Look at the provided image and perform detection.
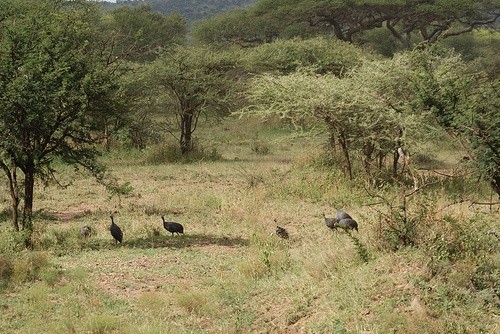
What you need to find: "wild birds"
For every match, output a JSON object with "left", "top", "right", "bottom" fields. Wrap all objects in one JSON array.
[
  {"left": 322, "top": 211, "right": 340, "bottom": 238},
  {"left": 109, "top": 215, "right": 123, "bottom": 245},
  {"left": 334, "top": 207, "right": 352, "bottom": 223},
  {"left": 274, "top": 218, "right": 289, "bottom": 241},
  {"left": 161, "top": 215, "right": 184, "bottom": 237},
  {"left": 334, "top": 218, "right": 358, "bottom": 236},
  {"left": 81, "top": 225, "right": 91, "bottom": 239}
]
